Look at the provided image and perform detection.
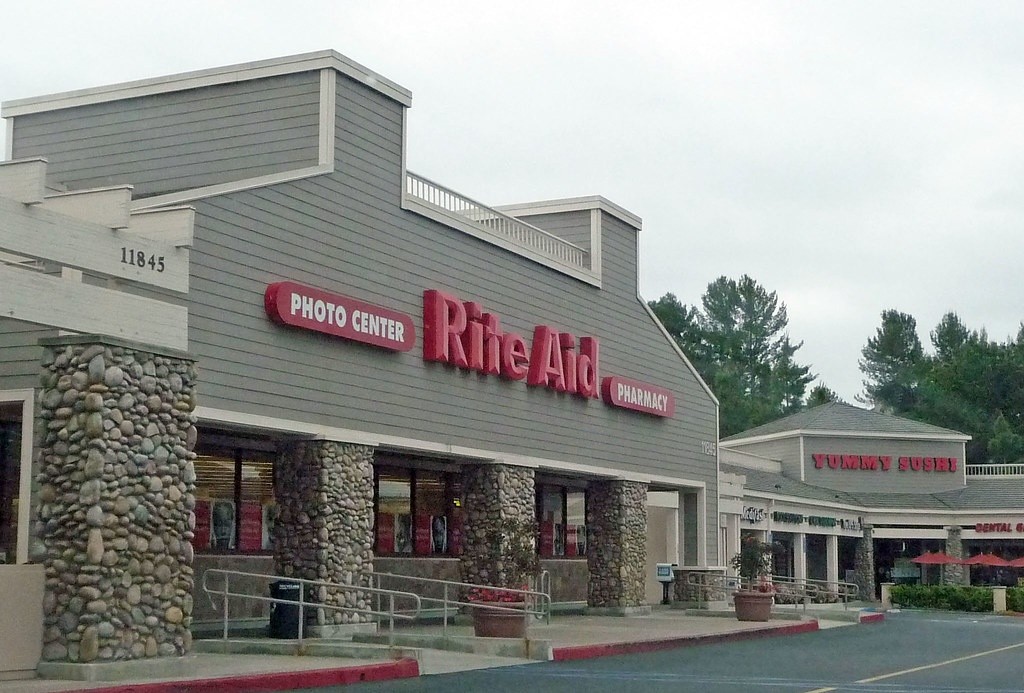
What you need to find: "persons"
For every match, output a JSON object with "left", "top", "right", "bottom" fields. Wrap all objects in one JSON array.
[
  {"left": 577, "top": 527, "right": 585, "bottom": 556},
  {"left": 212, "top": 502, "right": 234, "bottom": 549},
  {"left": 264, "top": 502, "right": 280, "bottom": 549},
  {"left": 554, "top": 524, "right": 564, "bottom": 554},
  {"left": 432, "top": 516, "right": 446, "bottom": 554},
  {"left": 396, "top": 514, "right": 411, "bottom": 551}
]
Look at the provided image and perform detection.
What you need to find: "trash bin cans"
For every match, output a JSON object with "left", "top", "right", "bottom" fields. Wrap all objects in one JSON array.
[{"left": 268, "top": 580, "right": 313, "bottom": 640}]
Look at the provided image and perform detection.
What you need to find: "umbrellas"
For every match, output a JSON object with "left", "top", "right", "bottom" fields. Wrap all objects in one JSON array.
[
  {"left": 961, "top": 554, "right": 1024, "bottom": 586},
  {"left": 910, "top": 551, "right": 962, "bottom": 584}
]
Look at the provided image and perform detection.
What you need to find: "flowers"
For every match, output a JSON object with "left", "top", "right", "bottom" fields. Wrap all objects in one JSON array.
[{"left": 470, "top": 582, "right": 530, "bottom": 602}]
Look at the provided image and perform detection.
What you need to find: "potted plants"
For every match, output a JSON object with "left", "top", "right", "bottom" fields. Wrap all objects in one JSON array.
[
  {"left": 473, "top": 517, "right": 542, "bottom": 638},
  {"left": 728, "top": 532, "right": 785, "bottom": 621}
]
